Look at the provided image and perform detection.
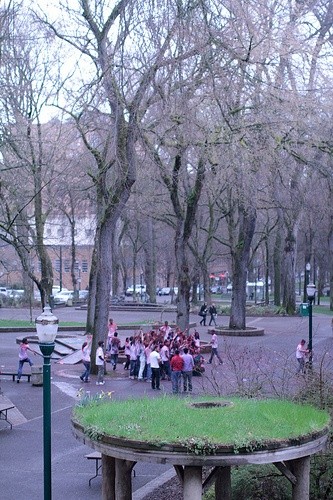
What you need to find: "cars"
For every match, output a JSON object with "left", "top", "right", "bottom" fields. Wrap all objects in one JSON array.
[
  {"left": 0, "top": 286, "right": 24, "bottom": 300},
  {"left": 158, "top": 287, "right": 179, "bottom": 296},
  {"left": 34, "top": 285, "right": 89, "bottom": 307}
]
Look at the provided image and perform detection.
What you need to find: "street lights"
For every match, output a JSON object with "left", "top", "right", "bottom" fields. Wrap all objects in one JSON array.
[
  {"left": 305, "top": 262, "right": 311, "bottom": 303},
  {"left": 306, "top": 280, "right": 316, "bottom": 377},
  {"left": 34, "top": 300, "right": 59, "bottom": 500}
]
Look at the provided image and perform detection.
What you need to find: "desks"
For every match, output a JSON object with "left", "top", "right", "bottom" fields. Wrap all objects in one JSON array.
[
  {"left": 85, "top": 450, "right": 135, "bottom": 486},
  {"left": 0, "top": 404, "right": 15, "bottom": 430}
]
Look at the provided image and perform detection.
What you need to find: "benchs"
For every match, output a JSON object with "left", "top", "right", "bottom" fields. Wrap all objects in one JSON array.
[{"left": 0, "top": 372, "right": 33, "bottom": 382}]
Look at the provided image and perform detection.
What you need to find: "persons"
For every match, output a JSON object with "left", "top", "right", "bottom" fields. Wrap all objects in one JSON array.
[
  {"left": 124, "top": 321, "right": 205, "bottom": 382},
  {"left": 200, "top": 302, "right": 208, "bottom": 326},
  {"left": 96, "top": 340, "right": 105, "bottom": 385},
  {"left": 16, "top": 338, "right": 37, "bottom": 383},
  {"left": 80, "top": 337, "right": 92, "bottom": 383},
  {"left": 149, "top": 346, "right": 162, "bottom": 390},
  {"left": 181, "top": 348, "right": 194, "bottom": 391},
  {"left": 209, "top": 303, "right": 217, "bottom": 326},
  {"left": 107, "top": 319, "right": 118, "bottom": 351},
  {"left": 170, "top": 351, "right": 185, "bottom": 395},
  {"left": 110, "top": 331, "right": 121, "bottom": 371},
  {"left": 295, "top": 339, "right": 309, "bottom": 375},
  {"left": 207, "top": 329, "right": 223, "bottom": 365}
]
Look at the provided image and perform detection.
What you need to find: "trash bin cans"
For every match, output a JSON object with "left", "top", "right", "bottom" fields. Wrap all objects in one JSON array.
[
  {"left": 299, "top": 303, "right": 309, "bottom": 316},
  {"left": 30, "top": 365, "right": 43, "bottom": 386}
]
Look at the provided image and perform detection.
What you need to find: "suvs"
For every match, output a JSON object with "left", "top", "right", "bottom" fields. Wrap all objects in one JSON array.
[{"left": 126, "top": 285, "right": 146, "bottom": 296}]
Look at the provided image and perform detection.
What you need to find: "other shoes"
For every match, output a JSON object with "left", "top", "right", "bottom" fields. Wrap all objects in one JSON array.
[
  {"left": 80, "top": 376, "right": 84, "bottom": 381},
  {"left": 17, "top": 379, "right": 20, "bottom": 383}
]
